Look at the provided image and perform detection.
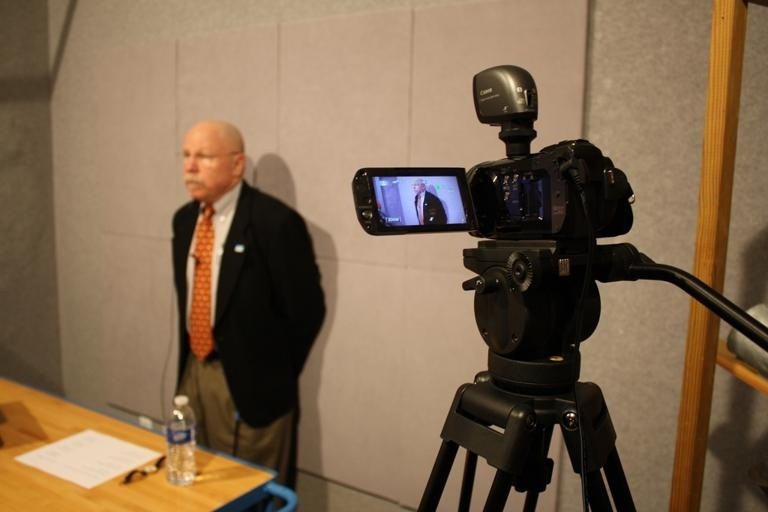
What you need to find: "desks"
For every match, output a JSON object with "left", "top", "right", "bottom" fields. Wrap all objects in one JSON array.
[{"left": 0, "top": 377, "right": 300, "bottom": 512}]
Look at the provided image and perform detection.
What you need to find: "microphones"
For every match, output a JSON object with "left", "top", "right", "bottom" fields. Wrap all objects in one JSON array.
[{"left": 193, "top": 255, "right": 200, "bottom": 267}]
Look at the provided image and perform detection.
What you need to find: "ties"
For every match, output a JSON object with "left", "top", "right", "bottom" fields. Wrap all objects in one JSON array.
[
  {"left": 417, "top": 195, "right": 423, "bottom": 225},
  {"left": 189, "top": 204, "right": 215, "bottom": 365}
]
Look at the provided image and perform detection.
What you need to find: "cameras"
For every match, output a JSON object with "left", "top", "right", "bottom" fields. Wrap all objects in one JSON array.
[{"left": 352, "top": 64, "right": 636, "bottom": 238}]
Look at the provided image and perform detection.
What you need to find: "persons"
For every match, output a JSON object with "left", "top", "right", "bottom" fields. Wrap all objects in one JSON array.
[
  {"left": 412, "top": 180, "right": 448, "bottom": 226},
  {"left": 162, "top": 118, "right": 330, "bottom": 496}
]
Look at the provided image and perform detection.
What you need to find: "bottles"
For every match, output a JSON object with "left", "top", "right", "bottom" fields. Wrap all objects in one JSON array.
[{"left": 166, "top": 394, "right": 196, "bottom": 487}]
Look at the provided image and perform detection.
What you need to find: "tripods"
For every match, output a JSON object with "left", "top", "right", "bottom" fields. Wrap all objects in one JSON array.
[{"left": 416, "top": 238, "right": 768, "bottom": 512}]
